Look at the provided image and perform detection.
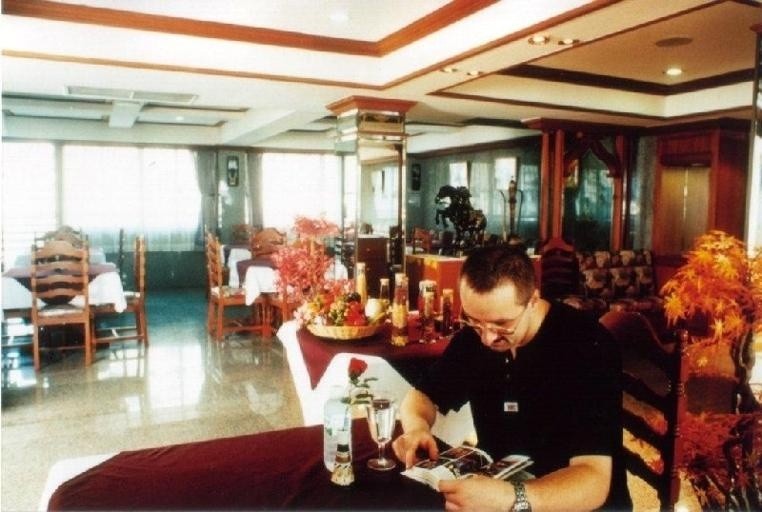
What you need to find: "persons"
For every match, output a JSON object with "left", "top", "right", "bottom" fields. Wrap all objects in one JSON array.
[{"left": 391, "top": 243, "right": 634, "bottom": 512}]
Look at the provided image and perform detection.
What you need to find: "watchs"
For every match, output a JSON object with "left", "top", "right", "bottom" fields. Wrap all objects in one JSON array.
[{"left": 507, "top": 480, "right": 533, "bottom": 511}]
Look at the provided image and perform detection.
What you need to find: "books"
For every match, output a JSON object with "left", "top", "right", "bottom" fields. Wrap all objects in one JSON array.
[{"left": 400, "top": 443, "right": 535, "bottom": 494}]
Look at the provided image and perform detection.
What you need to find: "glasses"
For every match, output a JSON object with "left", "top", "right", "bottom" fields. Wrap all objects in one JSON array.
[{"left": 457, "top": 297, "right": 524, "bottom": 334}]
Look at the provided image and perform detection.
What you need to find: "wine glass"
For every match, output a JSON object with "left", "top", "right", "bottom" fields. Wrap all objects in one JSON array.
[{"left": 362, "top": 393, "right": 398, "bottom": 472}]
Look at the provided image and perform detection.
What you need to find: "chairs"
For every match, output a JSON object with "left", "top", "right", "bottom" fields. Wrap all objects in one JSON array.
[
  {"left": 2, "top": 277, "right": 33, "bottom": 356},
  {"left": 34, "top": 228, "right": 90, "bottom": 260},
  {"left": 31, "top": 245, "right": 96, "bottom": 369},
  {"left": 605, "top": 310, "right": 680, "bottom": 511},
  {"left": 200, "top": 223, "right": 761, "bottom": 413},
  {"left": 92, "top": 238, "right": 147, "bottom": 353}
]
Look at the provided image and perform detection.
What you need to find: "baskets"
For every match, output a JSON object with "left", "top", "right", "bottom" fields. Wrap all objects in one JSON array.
[{"left": 307, "top": 324, "right": 377, "bottom": 340}]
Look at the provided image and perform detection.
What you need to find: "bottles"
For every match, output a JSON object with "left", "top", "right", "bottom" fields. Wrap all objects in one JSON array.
[
  {"left": 350, "top": 260, "right": 454, "bottom": 347},
  {"left": 322, "top": 385, "right": 354, "bottom": 473}
]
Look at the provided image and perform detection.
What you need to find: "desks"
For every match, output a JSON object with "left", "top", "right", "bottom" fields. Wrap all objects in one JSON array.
[
  {"left": 6, "top": 264, "right": 121, "bottom": 353},
  {"left": 46, "top": 417, "right": 452, "bottom": 510}
]
